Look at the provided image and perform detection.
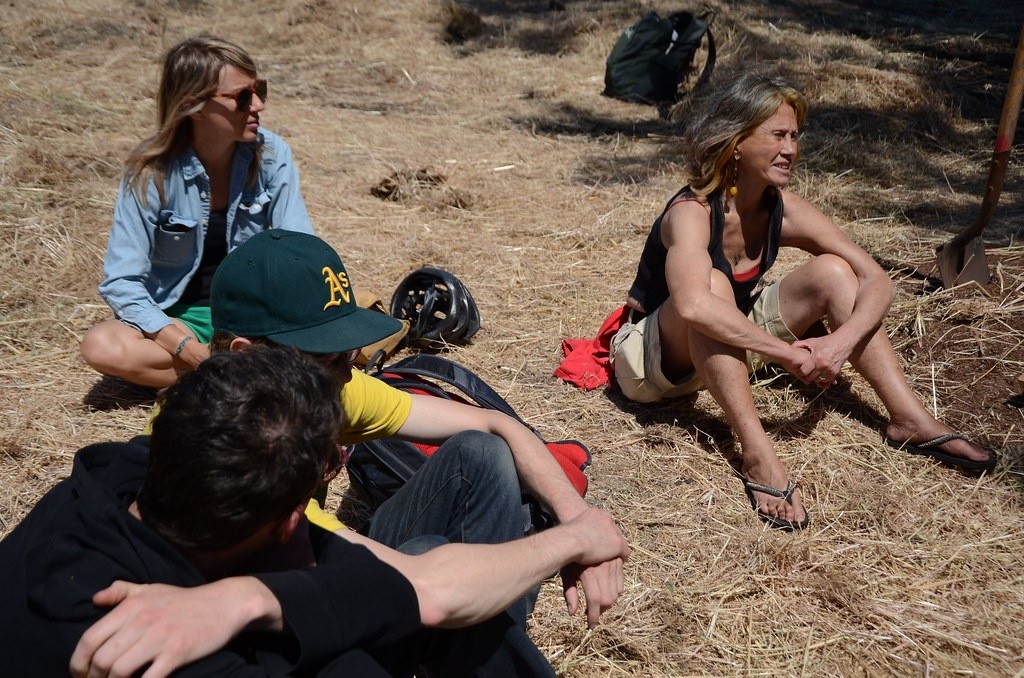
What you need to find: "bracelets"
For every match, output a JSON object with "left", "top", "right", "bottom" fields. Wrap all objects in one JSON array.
[{"left": 176, "top": 336, "right": 193, "bottom": 356}]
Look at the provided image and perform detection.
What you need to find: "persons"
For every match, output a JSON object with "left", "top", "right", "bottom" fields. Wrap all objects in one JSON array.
[
  {"left": 0, "top": 345, "right": 421, "bottom": 678},
  {"left": 134, "top": 228, "right": 632, "bottom": 678},
  {"left": 608, "top": 69, "right": 996, "bottom": 528},
  {"left": 80, "top": 32, "right": 318, "bottom": 395}
]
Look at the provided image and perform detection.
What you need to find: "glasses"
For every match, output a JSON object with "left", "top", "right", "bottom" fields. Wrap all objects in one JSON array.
[{"left": 206, "top": 79, "right": 268, "bottom": 111}]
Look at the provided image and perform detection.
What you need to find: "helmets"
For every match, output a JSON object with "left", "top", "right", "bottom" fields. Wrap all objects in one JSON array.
[{"left": 390, "top": 265, "right": 479, "bottom": 352}]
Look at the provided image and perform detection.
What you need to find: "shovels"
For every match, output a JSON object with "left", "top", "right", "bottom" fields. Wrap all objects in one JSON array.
[{"left": 935, "top": 23, "right": 1024, "bottom": 293}]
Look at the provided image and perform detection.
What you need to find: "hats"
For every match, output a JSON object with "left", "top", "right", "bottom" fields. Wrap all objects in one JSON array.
[{"left": 211, "top": 229, "right": 403, "bottom": 352}]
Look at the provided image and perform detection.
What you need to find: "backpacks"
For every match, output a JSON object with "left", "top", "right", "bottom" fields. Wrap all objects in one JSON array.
[
  {"left": 599, "top": 10, "right": 716, "bottom": 106},
  {"left": 346, "top": 355, "right": 592, "bottom": 518}
]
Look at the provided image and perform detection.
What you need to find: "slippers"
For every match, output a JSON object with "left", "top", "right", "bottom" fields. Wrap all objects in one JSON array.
[
  {"left": 741, "top": 474, "right": 809, "bottom": 532},
  {"left": 883, "top": 434, "right": 997, "bottom": 476}
]
